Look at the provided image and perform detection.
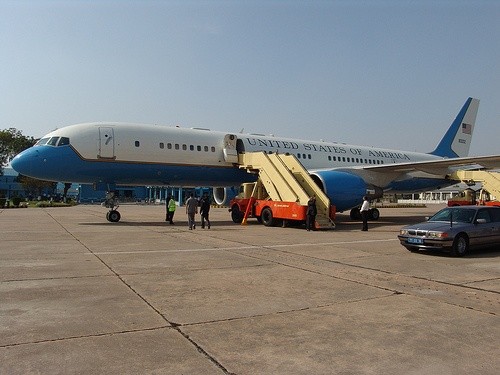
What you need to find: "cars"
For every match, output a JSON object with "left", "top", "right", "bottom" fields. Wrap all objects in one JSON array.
[{"left": 397, "top": 204, "right": 500, "bottom": 257}]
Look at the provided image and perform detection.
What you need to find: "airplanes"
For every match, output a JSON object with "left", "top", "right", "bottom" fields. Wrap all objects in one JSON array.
[{"left": 9, "top": 97, "right": 500, "bottom": 235}]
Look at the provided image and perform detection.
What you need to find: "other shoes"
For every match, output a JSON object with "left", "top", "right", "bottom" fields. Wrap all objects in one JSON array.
[
  {"left": 189, "top": 226, "right": 192, "bottom": 230},
  {"left": 201, "top": 226, "right": 205, "bottom": 229},
  {"left": 307, "top": 228, "right": 310, "bottom": 231},
  {"left": 208, "top": 226, "right": 210, "bottom": 229},
  {"left": 193, "top": 225, "right": 195, "bottom": 229},
  {"left": 361, "top": 229, "right": 368, "bottom": 231},
  {"left": 313, "top": 229, "right": 317, "bottom": 231}
]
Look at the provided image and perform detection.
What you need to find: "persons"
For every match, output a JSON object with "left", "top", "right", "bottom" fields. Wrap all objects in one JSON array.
[
  {"left": 168, "top": 196, "right": 175, "bottom": 224},
  {"left": 186, "top": 193, "right": 198, "bottom": 230},
  {"left": 185, "top": 193, "right": 208, "bottom": 206},
  {"left": 307, "top": 197, "right": 317, "bottom": 231},
  {"left": 359, "top": 196, "right": 369, "bottom": 232},
  {"left": 200, "top": 199, "right": 210, "bottom": 229},
  {"left": 165, "top": 195, "right": 171, "bottom": 221},
  {"left": 36, "top": 191, "right": 65, "bottom": 202}
]
